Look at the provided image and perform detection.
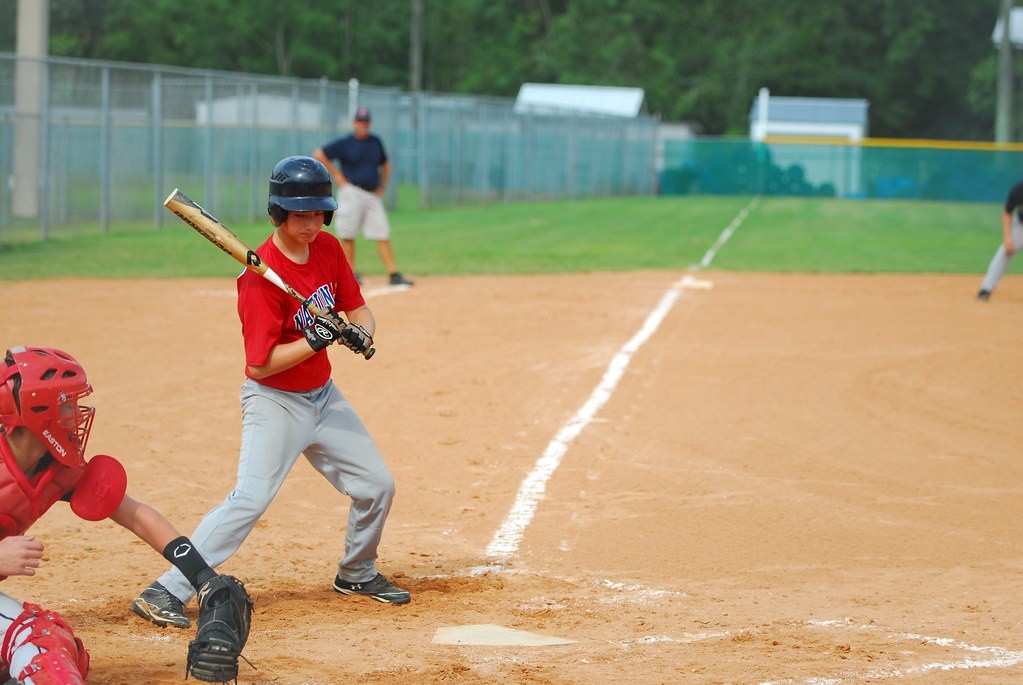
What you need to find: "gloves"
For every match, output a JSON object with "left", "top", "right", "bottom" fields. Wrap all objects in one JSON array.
[
  {"left": 337, "top": 322, "right": 374, "bottom": 355},
  {"left": 304, "top": 305, "right": 348, "bottom": 353}
]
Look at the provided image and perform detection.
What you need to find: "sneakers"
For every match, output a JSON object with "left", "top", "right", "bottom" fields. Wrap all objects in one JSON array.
[
  {"left": 131, "top": 583, "right": 190, "bottom": 628},
  {"left": 332, "top": 572, "right": 410, "bottom": 603}
]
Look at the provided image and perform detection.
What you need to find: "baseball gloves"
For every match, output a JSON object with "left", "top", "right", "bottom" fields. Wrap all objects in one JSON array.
[{"left": 183, "top": 566, "right": 259, "bottom": 685}]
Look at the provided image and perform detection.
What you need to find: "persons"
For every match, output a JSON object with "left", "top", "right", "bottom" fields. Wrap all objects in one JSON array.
[
  {"left": 132, "top": 154, "right": 411, "bottom": 629},
  {"left": 313, "top": 104, "right": 414, "bottom": 287},
  {"left": 0, "top": 341, "right": 253, "bottom": 685},
  {"left": 976, "top": 177, "right": 1023, "bottom": 302}
]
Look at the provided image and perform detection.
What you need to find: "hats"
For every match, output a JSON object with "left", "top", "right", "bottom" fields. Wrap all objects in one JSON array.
[{"left": 356, "top": 108, "right": 370, "bottom": 120}]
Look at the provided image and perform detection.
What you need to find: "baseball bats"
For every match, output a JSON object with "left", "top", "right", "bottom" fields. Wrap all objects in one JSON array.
[{"left": 163, "top": 186, "right": 377, "bottom": 361}]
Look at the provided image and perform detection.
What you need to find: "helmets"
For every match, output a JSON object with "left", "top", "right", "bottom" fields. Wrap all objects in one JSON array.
[
  {"left": 0, "top": 346, "right": 95, "bottom": 467},
  {"left": 268, "top": 156, "right": 339, "bottom": 227}
]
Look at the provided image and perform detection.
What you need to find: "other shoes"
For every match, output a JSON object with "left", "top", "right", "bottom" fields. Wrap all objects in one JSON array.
[
  {"left": 979, "top": 290, "right": 989, "bottom": 299},
  {"left": 389, "top": 275, "right": 412, "bottom": 285},
  {"left": 353, "top": 272, "right": 362, "bottom": 285}
]
